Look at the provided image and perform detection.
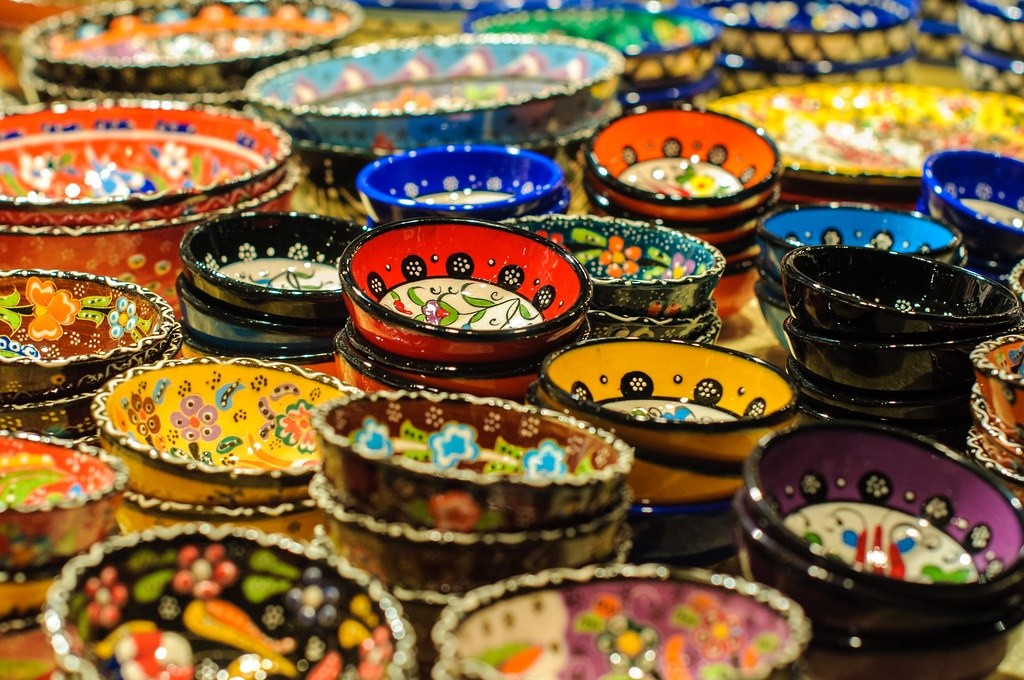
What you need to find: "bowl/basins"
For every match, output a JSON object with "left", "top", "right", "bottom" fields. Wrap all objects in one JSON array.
[{"left": 0, "top": 0, "right": 1024, "bottom": 680}]
